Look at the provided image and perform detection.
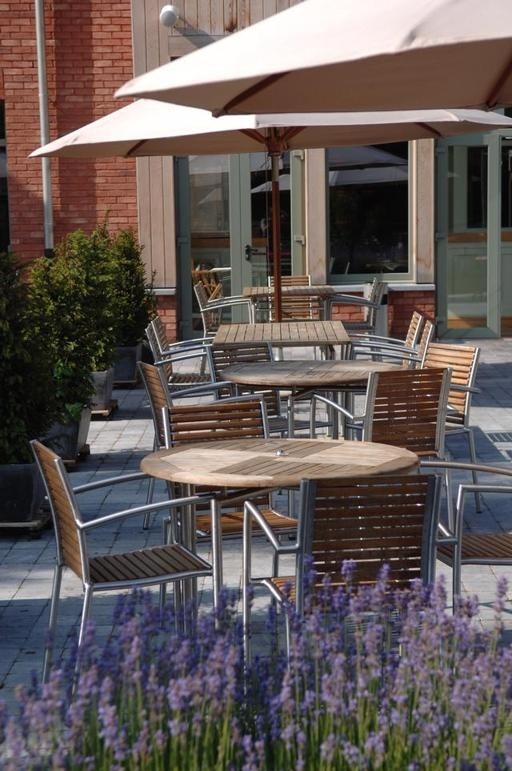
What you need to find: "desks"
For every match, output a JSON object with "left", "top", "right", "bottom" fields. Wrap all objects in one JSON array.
[{"left": 140, "top": 435, "right": 418, "bottom": 658}]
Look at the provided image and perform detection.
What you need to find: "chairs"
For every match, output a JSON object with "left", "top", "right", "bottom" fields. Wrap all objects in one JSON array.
[
  {"left": 161, "top": 400, "right": 298, "bottom": 639},
  {"left": 137, "top": 264, "right": 481, "bottom": 527},
  {"left": 29, "top": 440, "right": 216, "bottom": 706},
  {"left": 419, "top": 461, "right": 512, "bottom": 620},
  {"left": 238, "top": 473, "right": 444, "bottom": 702}
]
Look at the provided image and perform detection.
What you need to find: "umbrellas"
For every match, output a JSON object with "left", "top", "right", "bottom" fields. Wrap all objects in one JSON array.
[
  {"left": 189, "top": 144, "right": 457, "bottom": 209},
  {"left": 27, "top": 98, "right": 511, "bottom": 362},
  {"left": 115, "top": 0, "right": 512, "bottom": 113}
]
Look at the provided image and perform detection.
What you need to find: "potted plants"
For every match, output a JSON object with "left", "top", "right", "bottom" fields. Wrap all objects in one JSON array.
[{"left": 0, "top": 206, "right": 160, "bottom": 523}]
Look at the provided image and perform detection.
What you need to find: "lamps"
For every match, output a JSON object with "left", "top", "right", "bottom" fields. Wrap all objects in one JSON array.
[{"left": 160, "top": 5, "right": 178, "bottom": 28}]
